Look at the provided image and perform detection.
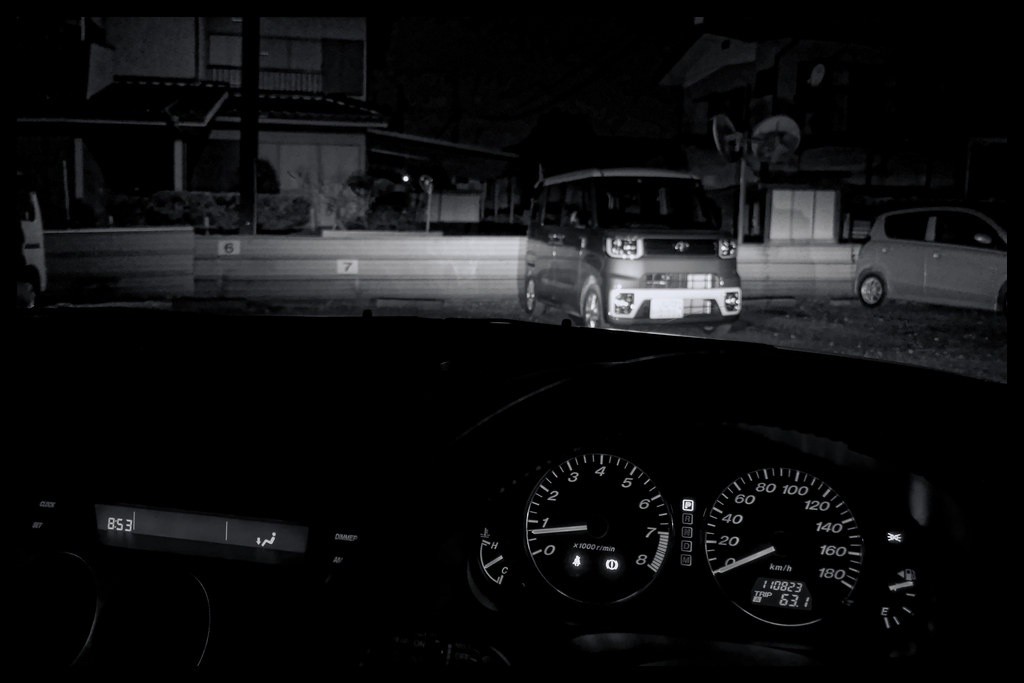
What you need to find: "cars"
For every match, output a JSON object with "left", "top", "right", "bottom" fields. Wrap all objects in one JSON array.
[{"left": 852, "top": 202, "right": 1008, "bottom": 319}]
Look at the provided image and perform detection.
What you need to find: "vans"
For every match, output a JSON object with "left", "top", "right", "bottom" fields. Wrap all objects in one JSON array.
[{"left": 521, "top": 167, "right": 748, "bottom": 333}]
